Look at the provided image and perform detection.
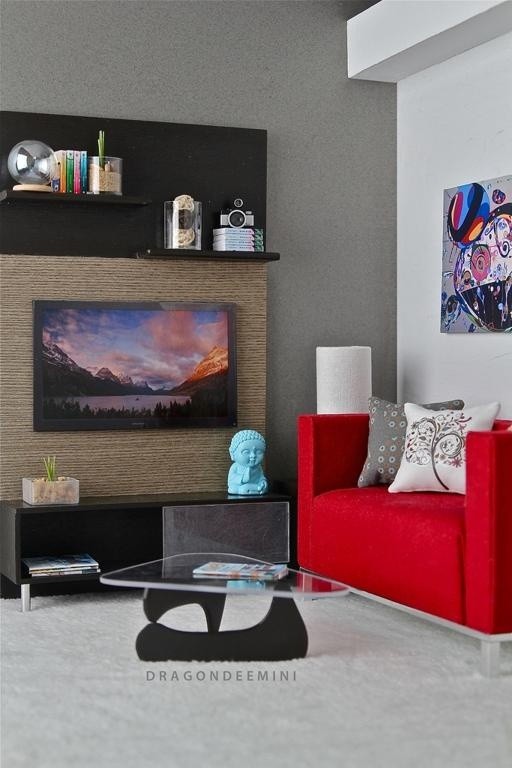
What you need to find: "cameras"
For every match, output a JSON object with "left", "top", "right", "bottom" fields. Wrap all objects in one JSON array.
[{"left": 219, "top": 199, "right": 255, "bottom": 228}]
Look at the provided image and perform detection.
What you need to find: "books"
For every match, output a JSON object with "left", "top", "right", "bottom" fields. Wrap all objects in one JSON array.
[
  {"left": 212, "top": 226, "right": 265, "bottom": 252},
  {"left": 49, "top": 149, "right": 89, "bottom": 194},
  {"left": 193, "top": 561, "right": 288, "bottom": 578},
  {"left": 22, "top": 552, "right": 103, "bottom": 578}
]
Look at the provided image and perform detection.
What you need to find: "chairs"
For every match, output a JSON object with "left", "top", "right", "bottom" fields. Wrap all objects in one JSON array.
[{"left": 298, "top": 413, "right": 512, "bottom": 634}]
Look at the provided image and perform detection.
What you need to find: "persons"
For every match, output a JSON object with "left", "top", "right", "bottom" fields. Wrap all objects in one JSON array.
[{"left": 227, "top": 429, "right": 268, "bottom": 495}]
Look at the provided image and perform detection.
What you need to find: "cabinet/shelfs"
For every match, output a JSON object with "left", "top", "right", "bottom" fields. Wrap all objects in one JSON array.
[{"left": 0, "top": 111, "right": 296, "bottom": 612}]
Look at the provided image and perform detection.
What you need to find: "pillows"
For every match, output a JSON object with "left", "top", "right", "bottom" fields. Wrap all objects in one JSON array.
[{"left": 356, "top": 397, "right": 501, "bottom": 496}]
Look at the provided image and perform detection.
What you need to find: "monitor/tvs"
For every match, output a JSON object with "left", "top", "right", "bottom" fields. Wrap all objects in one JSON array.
[{"left": 32, "top": 300, "right": 236, "bottom": 431}]
